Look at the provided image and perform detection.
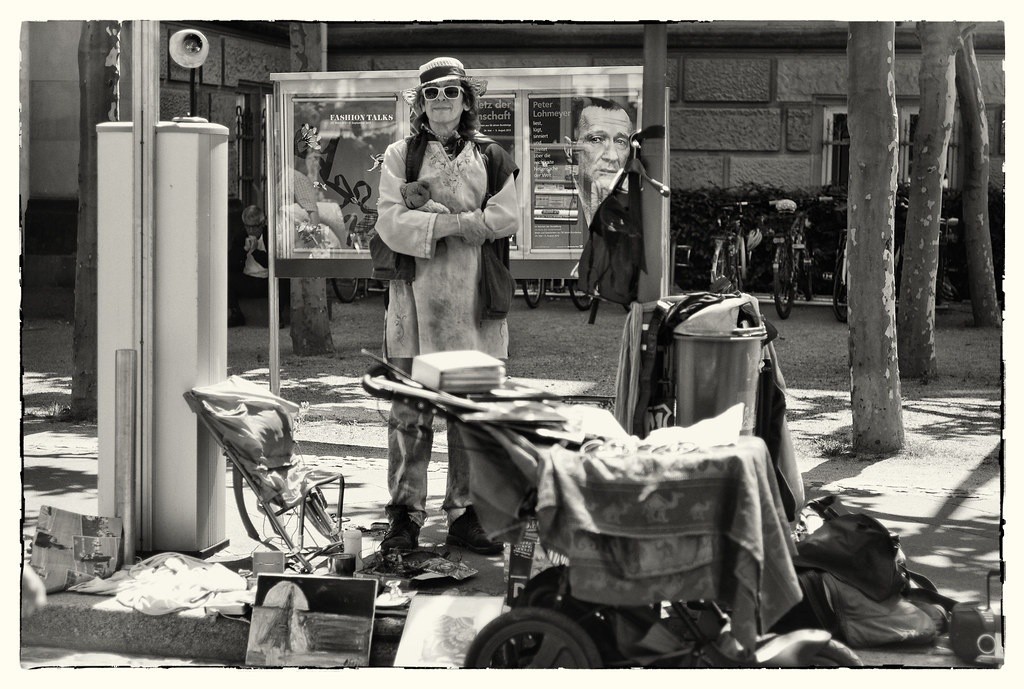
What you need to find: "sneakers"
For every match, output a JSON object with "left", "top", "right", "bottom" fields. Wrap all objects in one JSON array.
[
  {"left": 446, "top": 506, "right": 505, "bottom": 556},
  {"left": 378, "top": 503, "right": 420, "bottom": 556}
]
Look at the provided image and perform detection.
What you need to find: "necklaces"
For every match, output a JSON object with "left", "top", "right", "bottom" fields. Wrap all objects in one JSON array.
[{"left": 436, "top": 134, "right": 448, "bottom": 139}]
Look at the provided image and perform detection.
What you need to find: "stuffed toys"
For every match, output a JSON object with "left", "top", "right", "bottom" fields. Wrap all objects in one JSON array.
[{"left": 399, "top": 181, "right": 450, "bottom": 259}]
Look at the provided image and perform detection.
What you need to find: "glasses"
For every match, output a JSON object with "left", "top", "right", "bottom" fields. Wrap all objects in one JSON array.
[{"left": 421, "top": 85, "right": 466, "bottom": 102}]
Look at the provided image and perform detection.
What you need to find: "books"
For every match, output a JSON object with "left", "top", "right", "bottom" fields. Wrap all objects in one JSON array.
[{"left": 411, "top": 349, "right": 506, "bottom": 393}]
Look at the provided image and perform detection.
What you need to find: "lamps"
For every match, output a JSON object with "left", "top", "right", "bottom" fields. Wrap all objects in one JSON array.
[{"left": 169, "top": 29, "right": 210, "bottom": 122}]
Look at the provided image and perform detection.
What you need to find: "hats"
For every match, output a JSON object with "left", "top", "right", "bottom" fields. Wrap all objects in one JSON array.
[{"left": 401, "top": 57, "right": 488, "bottom": 106}]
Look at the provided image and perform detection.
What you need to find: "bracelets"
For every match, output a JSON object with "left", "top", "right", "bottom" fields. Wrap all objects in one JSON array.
[{"left": 456, "top": 213, "right": 461, "bottom": 236}]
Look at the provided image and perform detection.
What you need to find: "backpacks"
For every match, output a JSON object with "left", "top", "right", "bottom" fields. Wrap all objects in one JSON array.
[{"left": 577, "top": 131, "right": 648, "bottom": 324}]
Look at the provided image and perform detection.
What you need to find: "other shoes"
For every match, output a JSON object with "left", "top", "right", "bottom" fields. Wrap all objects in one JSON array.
[{"left": 228, "top": 313, "right": 248, "bottom": 329}]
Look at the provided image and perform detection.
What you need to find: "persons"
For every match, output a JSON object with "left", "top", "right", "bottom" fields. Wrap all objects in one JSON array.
[
  {"left": 228, "top": 205, "right": 287, "bottom": 328},
  {"left": 375, "top": 57, "right": 520, "bottom": 555},
  {"left": 535, "top": 98, "right": 634, "bottom": 245}
]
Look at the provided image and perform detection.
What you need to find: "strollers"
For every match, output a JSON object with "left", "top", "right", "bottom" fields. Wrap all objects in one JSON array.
[{"left": 361, "top": 361, "right": 864, "bottom": 672}]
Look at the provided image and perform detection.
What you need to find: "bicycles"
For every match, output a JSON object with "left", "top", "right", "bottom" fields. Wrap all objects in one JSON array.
[
  {"left": 823, "top": 182, "right": 959, "bottom": 322},
  {"left": 762, "top": 196, "right": 824, "bottom": 321},
  {"left": 329, "top": 275, "right": 594, "bottom": 313},
  {"left": 705, "top": 197, "right": 765, "bottom": 295}
]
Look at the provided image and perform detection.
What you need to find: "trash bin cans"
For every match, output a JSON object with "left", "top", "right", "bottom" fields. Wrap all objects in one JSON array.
[{"left": 674, "top": 290, "right": 767, "bottom": 437}]
